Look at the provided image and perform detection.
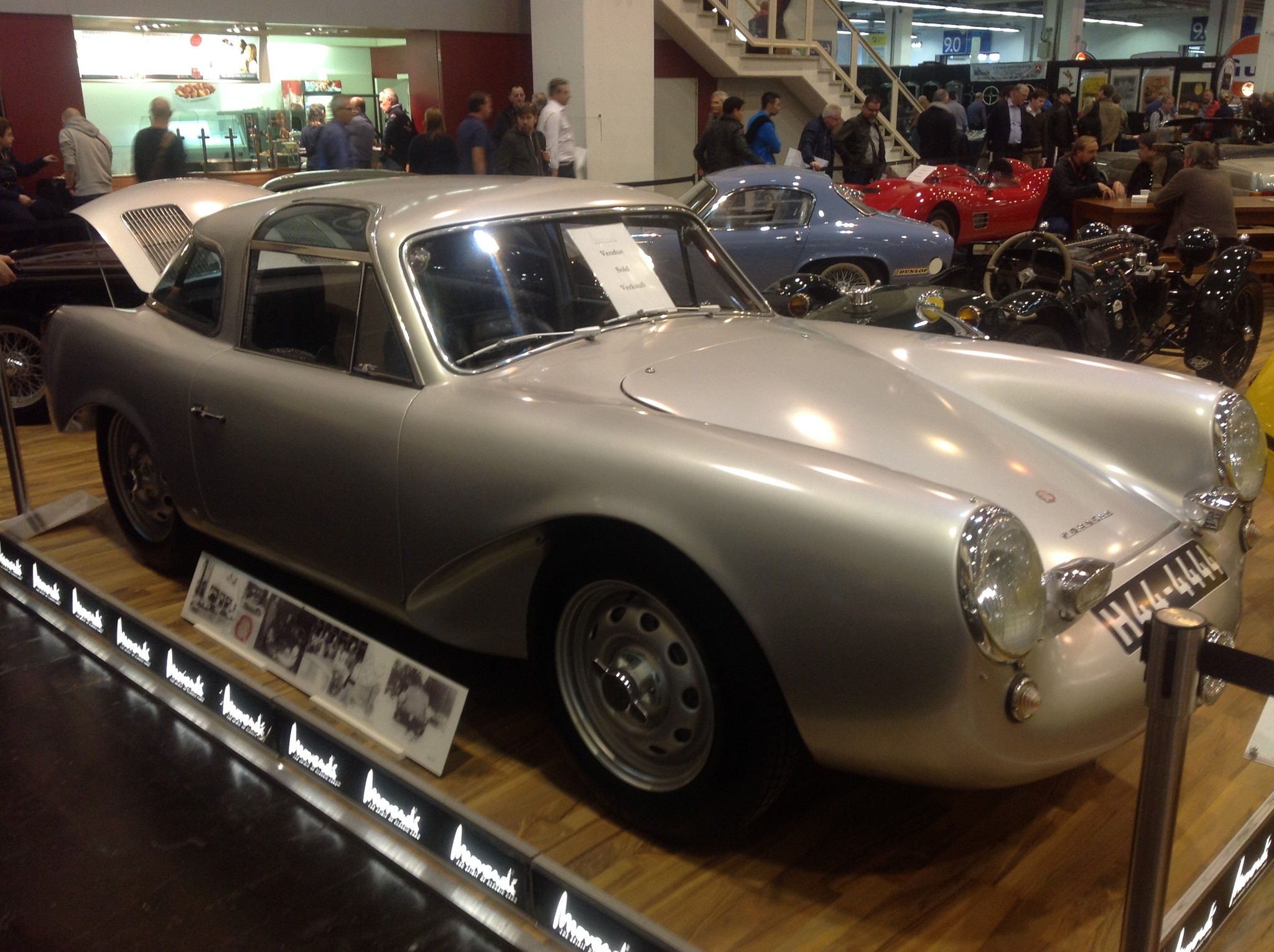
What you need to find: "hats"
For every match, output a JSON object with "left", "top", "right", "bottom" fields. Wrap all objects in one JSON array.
[
  {"left": 1219, "top": 93, "right": 1229, "bottom": 100},
  {"left": 1056, "top": 86, "right": 1075, "bottom": 97}
]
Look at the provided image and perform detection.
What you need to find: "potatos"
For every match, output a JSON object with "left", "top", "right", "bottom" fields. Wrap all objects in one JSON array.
[{"left": 176, "top": 82, "right": 215, "bottom": 98}]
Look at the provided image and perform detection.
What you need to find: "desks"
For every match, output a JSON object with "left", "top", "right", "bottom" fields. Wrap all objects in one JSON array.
[{"left": 1071, "top": 195, "right": 1274, "bottom": 235}]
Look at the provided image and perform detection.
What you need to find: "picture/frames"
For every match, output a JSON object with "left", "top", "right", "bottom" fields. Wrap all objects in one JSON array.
[
  {"left": 1176, "top": 71, "right": 1213, "bottom": 120},
  {"left": 1138, "top": 65, "right": 1176, "bottom": 113},
  {"left": 1076, "top": 68, "right": 1109, "bottom": 114},
  {"left": 1109, "top": 66, "right": 1141, "bottom": 113},
  {"left": 1057, "top": 66, "right": 1080, "bottom": 97}
]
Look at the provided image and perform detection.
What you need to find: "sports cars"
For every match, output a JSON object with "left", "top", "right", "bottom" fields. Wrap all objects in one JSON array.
[
  {"left": 1088, "top": 116, "right": 1274, "bottom": 197},
  {"left": 837, "top": 159, "right": 1054, "bottom": 250}
]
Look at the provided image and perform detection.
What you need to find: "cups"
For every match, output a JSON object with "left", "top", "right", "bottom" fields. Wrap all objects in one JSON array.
[
  {"left": 1140, "top": 190, "right": 1150, "bottom": 196},
  {"left": 286, "top": 147, "right": 300, "bottom": 169}
]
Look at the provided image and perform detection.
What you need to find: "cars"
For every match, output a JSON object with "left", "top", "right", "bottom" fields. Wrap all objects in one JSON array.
[
  {"left": 627, "top": 165, "right": 957, "bottom": 315},
  {"left": 39, "top": 171, "right": 1267, "bottom": 823},
  {"left": 803, "top": 223, "right": 1264, "bottom": 390}
]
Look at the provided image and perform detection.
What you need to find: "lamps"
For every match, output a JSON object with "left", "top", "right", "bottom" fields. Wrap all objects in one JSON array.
[{"left": 1075, "top": 50, "right": 1110, "bottom": 74}]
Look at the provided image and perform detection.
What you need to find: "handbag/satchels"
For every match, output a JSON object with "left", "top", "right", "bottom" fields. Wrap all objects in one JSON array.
[
  {"left": 1077, "top": 101, "right": 1103, "bottom": 146},
  {"left": 871, "top": 160, "right": 886, "bottom": 181}
]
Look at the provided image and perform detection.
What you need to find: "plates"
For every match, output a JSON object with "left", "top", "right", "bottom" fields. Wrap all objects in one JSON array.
[{"left": 176, "top": 93, "right": 210, "bottom": 102}]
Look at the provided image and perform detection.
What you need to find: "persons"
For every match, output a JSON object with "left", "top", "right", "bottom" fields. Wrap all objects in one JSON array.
[
  {"left": 0, "top": 108, "right": 113, "bottom": 285},
  {"left": 835, "top": 96, "right": 886, "bottom": 185},
  {"left": 133, "top": 97, "right": 187, "bottom": 183},
  {"left": 909, "top": 84, "right": 1274, "bottom": 255},
  {"left": 693, "top": 90, "right": 782, "bottom": 175},
  {"left": 797, "top": 103, "right": 842, "bottom": 179},
  {"left": 702, "top": 0, "right": 791, "bottom": 53},
  {"left": 301, "top": 77, "right": 578, "bottom": 178}
]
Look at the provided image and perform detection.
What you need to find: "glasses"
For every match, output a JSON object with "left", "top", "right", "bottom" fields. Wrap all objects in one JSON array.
[
  {"left": 865, "top": 106, "right": 879, "bottom": 112},
  {"left": 339, "top": 107, "right": 352, "bottom": 111},
  {"left": 1182, "top": 155, "right": 1192, "bottom": 161}
]
